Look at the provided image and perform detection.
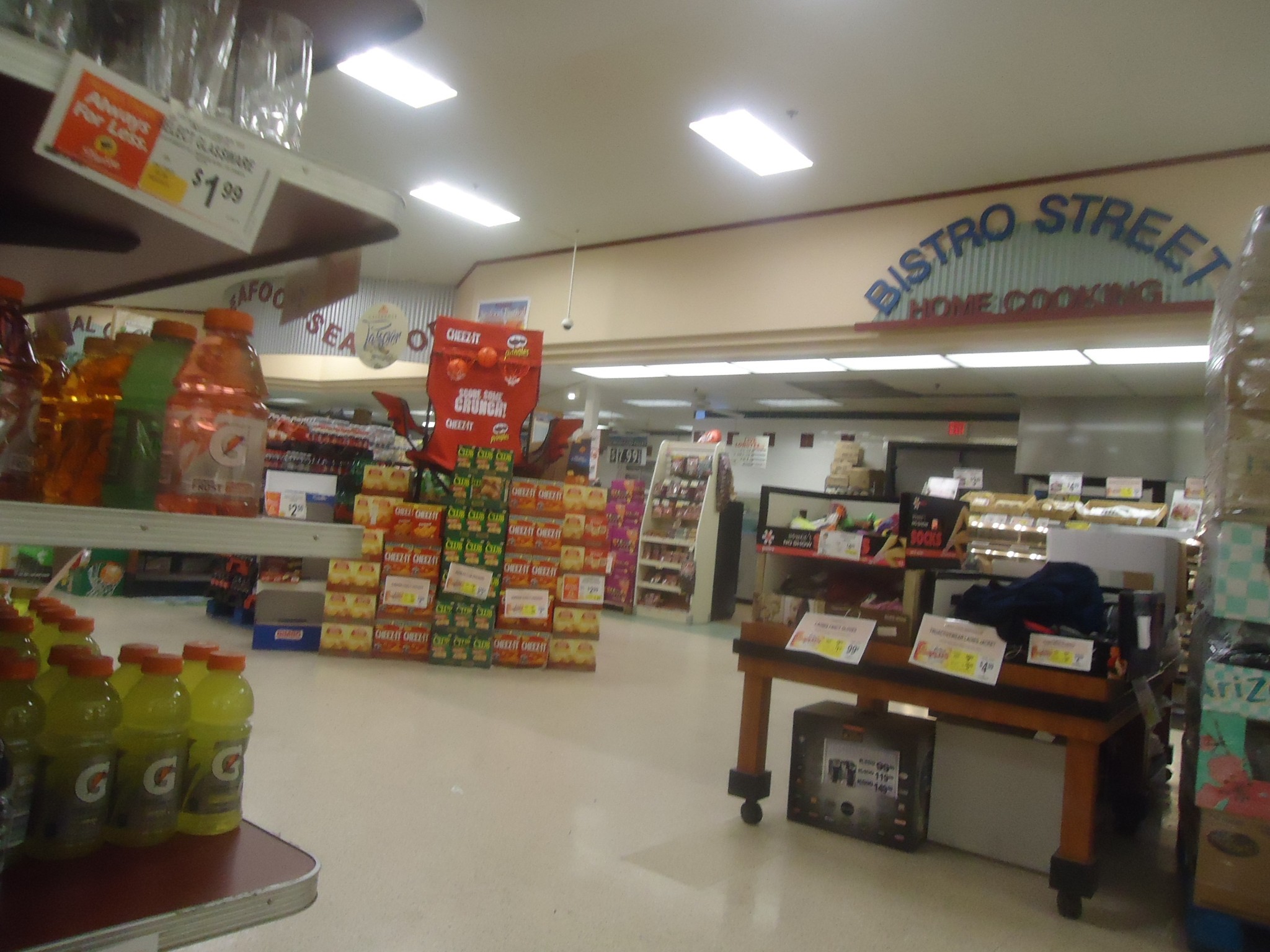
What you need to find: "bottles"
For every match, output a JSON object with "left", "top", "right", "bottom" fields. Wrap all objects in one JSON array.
[
  {"left": 46, "top": 336, "right": 113, "bottom": 499},
  {"left": 258, "top": 412, "right": 420, "bottom": 526},
  {"left": 155, "top": 307, "right": 269, "bottom": 518},
  {"left": 36, "top": 337, "right": 71, "bottom": 494},
  {"left": 83, "top": 332, "right": 153, "bottom": 507},
  {"left": 100, "top": 319, "right": 198, "bottom": 512},
  {"left": 0, "top": 276, "right": 44, "bottom": 503},
  {"left": 0, "top": 554, "right": 258, "bottom": 866}
]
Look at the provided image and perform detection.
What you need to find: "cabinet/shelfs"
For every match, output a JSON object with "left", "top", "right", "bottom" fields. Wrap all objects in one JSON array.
[
  {"left": 632, "top": 439, "right": 727, "bottom": 626},
  {"left": 0, "top": 0, "right": 422, "bottom": 952},
  {"left": 753, "top": 486, "right": 928, "bottom": 634}
]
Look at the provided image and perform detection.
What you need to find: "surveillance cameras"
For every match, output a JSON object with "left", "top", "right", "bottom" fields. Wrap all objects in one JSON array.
[{"left": 562, "top": 318, "right": 574, "bottom": 331}]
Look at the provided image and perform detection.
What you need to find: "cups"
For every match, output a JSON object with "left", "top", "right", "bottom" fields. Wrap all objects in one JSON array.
[
  {"left": 1, "top": 0, "right": 241, "bottom": 116},
  {"left": 232, "top": 8, "right": 314, "bottom": 154}
]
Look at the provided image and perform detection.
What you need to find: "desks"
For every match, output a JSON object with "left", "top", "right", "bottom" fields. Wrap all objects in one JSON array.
[{"left": 728, "top": 621, "right": 1178, "bottom": 919}]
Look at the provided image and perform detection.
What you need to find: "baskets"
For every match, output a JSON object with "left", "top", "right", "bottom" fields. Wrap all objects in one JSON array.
[{"left": 960, "top": 490, "right": 1167, "bottom": 526}]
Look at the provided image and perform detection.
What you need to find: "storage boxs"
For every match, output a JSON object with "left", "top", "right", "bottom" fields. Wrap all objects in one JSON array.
[
  {"left": 1192, "top": 807, "right": 1270, "bottom": 923},
  {"left": 824, "top": 441, "right": 884, "bottom": 499},
  {"left": 786, "top": 700, "right": 936, "bottom": 853},
  {"left": 252, "top": 446, "right": 646, "bottom": 674}
]
